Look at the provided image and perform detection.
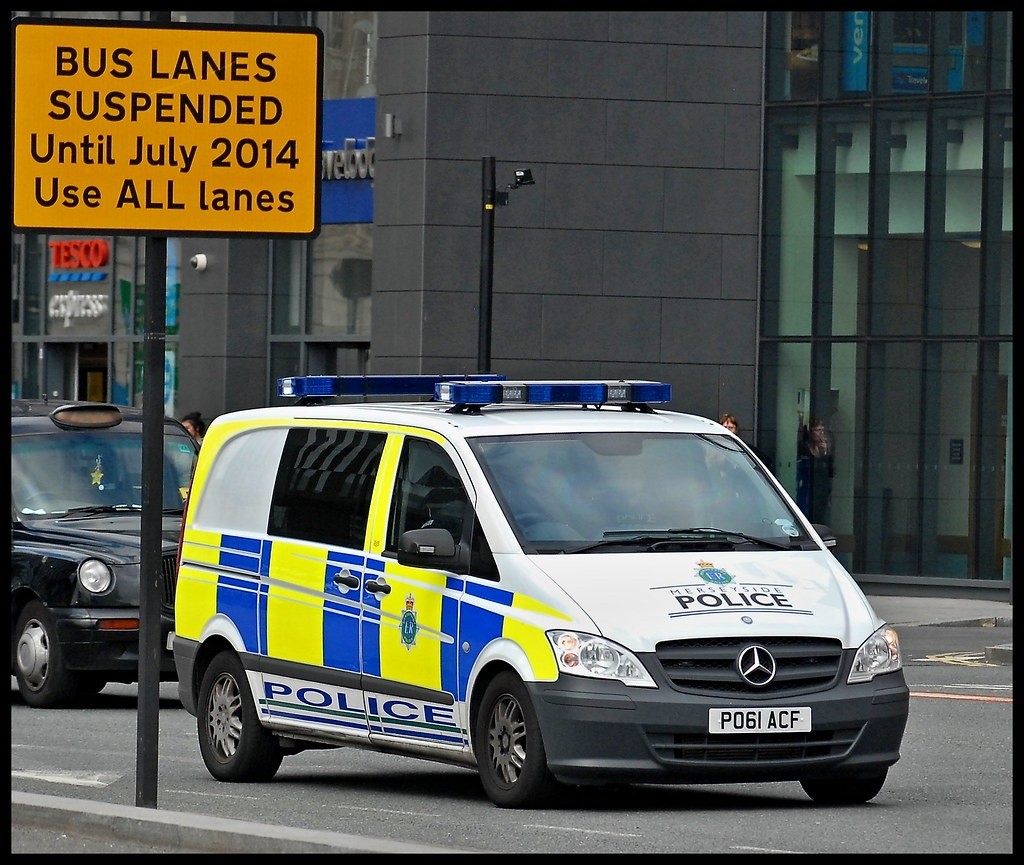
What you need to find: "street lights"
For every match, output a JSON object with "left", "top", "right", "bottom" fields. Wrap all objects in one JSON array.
[{"left": 476, "top": 168, "right": 537, "bottom": 373}]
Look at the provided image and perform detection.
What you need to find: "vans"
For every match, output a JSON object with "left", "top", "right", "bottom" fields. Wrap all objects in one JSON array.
[{"left": 169, "top": 371, "right": 908, "bottom": 808}]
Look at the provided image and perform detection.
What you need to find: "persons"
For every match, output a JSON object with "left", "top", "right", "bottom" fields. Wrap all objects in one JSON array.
[
  {"left": 795, "top": 418, "right": 830, "bottom": 528},
  {"left": 717, "top": 414, "right": 738, "bottom": 434},
  {"left": 179, "top": 411, "right": 205, "bottom": 446}
]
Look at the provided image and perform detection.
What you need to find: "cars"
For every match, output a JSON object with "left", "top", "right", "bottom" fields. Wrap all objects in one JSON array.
[{"left": 11, "top": 400, "right": 202, "bottom": 705}]
